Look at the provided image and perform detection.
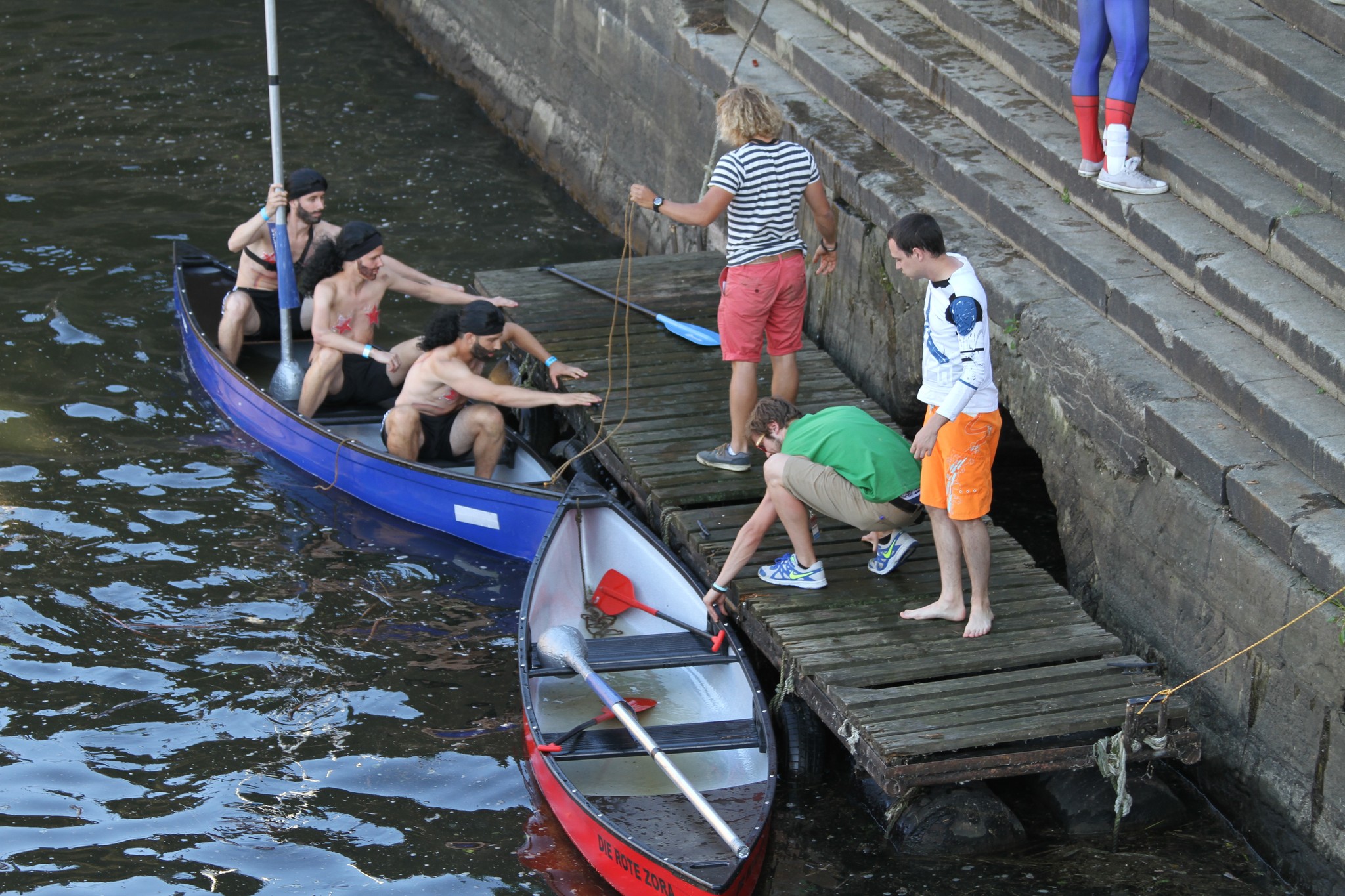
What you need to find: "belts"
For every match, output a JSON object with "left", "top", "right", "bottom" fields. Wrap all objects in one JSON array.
[{"left": 889, "top": 497, "right": 925, "bottom": 514}]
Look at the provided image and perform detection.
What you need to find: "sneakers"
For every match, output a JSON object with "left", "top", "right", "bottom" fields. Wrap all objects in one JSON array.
[
  {"left": 697, "top": 443, "right": 751, "bottom": 471},
  {"left": 758, "top": 554, "right": 829, "bottom": 590},
  {"left": 1078, "top": 160, "right": 1103, "bottom": 177},
  {"left": 1098, "top": 162, "right": 1169, "bottom": 194},
  {"left": 867, "top": 529, "right": 919, "bottom": 575}
]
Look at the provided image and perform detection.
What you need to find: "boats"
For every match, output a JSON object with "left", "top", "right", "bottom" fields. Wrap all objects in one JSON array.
[
  {"left": 518, "top": 468, "right": 782, "bottom": 896},
  {"left": 169, "top": 237, "right": 573, "bottom": 567}
]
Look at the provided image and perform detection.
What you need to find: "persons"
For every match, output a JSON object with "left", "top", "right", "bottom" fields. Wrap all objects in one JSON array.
[
  {"left": 297, "top": 221, "right": 519, "bottom": 420},
  {"left": 1069, "top": 0, "right": 1168, "bottom": 194},
  {"left": 630, "top": 86, "right": 841, "bottom": 472},
  {"left": 381, "top": 300, "right": 604, "bottom": 481},
  {"left": 218, "top": 169, "right": 464, "bottom": 364},
  {"left": 701, "top": 397, "right": 924, "bottom": 624},
  {"left": 885, "top": 214, "right": 1004, "bottom": 638}
]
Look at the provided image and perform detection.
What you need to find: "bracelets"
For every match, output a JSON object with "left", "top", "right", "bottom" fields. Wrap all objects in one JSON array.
[
  {"left": 820, "top": 238, "right": 838, "bottom": 252},
  {"left": 261, "top": 207, "right": 270, "bottom": 221},
  {"left": 362, "top": 344, "right": 372, "bottom": 357},
  {"left": 710, "top": 583, "right": 728, "bottom": 594},
  {"left": 544, "top": 356, "right": 557, "bottom": 367}
]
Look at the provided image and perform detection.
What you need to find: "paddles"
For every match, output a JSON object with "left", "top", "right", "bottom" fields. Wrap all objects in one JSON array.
[
  {"left": 590, "top": 568, "right": 725, "bottom": 651},
  {"left": 536, "top": 698, "right": 657, "bottom": 751},
  {"left": 538, "top": 262, "right": 721, "bottom": 346}
]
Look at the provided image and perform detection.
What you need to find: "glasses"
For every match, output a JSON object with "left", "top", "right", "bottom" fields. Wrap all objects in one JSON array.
[{"left": 755, "top": 432, "right": 767, "bottom": 452}]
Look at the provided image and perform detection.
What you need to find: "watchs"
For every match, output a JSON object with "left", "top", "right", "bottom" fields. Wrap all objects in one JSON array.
[{"left": 652, "top": 196, "right": 665, "bottom": 213}]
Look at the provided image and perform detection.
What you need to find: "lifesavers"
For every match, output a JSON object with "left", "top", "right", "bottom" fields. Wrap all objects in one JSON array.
[
  {"left": 517, "top": 406, "right": 551, "bottom": 452},
  {"left": 762, "top": 677, "right": 824, "bottom": 781},
  {"left": 550, "top": 438, "right": 601, "bottom": 484}
]
